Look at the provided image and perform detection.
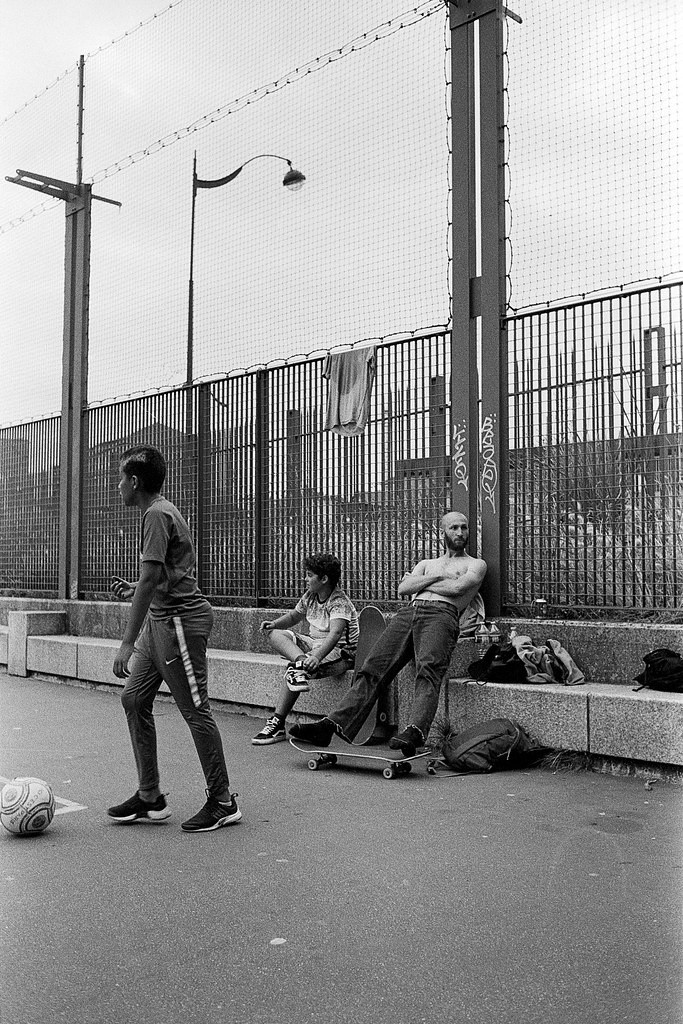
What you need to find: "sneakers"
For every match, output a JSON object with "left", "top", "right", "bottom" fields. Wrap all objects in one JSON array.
[
  {"left": 108, "top": 790, "right": 172, "bottom": 822},
  {"left": 182, "top": 789, "right": 243, "bottom": 832},
  {"left": 283, "top": 661, "right": 310, "bottom": 692},
  {"left": 253, "top": 716, "right": 286, "bottom": 744}
]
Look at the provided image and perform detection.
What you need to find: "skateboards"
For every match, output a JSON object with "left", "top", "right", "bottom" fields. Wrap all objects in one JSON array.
[
  {"left": 351, "top": 605, "right": 387, "bottom": 746},
  {"left": 289, "top": 737, "right": 433, "bottom": 779}
]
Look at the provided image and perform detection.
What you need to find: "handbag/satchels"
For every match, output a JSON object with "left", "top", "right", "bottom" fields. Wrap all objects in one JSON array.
[
  {"left": 467, "top": 643, "right": 531, "bottom": 685},
  {"left": 632, "top": 648, "right": 683, "bottom": 693}
]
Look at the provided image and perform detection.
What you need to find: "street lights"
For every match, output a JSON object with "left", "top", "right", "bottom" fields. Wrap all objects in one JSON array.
[{"left": 180, "top": 149, "right": 306, "bottom": 476}]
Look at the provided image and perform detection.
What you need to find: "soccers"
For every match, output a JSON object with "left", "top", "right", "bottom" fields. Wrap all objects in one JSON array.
[{"left": 0, "top": 776, "right": 56, "bottom": 836}]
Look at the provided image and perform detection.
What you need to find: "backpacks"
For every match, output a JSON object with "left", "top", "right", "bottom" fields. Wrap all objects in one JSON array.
[{"left": 435, "top": 718, "right": 548, "bottom": 778}]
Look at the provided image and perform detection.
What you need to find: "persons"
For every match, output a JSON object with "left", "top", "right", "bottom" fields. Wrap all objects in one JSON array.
[
  {"left": 288, "top": 511, "right": 487, "bottom": 756},
  {"left": 250, "top": 553, "right": 359, "bottom": 746},
  {"left": 108, "top": 446, "right": 241, "bottom": 832}
]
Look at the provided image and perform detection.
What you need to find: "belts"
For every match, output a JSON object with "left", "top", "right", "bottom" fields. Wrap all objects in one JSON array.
[{"left": 409, "top": 601, "right": 457, "bottom": 615}]
